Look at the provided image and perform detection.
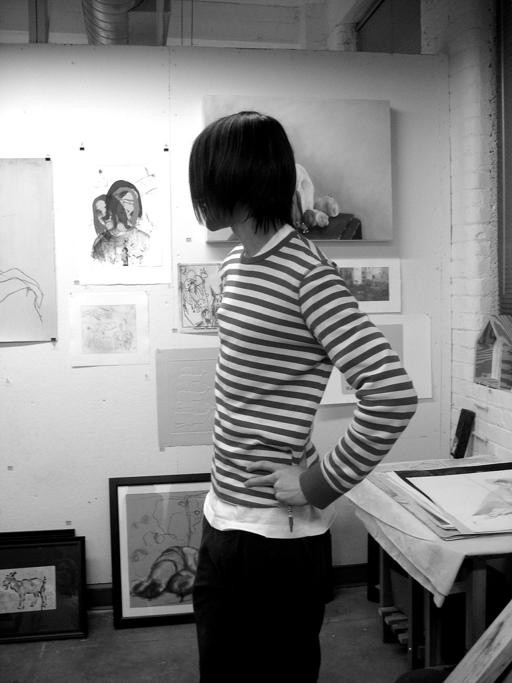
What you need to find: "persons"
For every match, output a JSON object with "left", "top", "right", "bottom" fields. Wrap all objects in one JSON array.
[
  {"left": 92, "top": 181, "right": 149, "bottom": 262},
  {"left": 190, "top": 112, "right": 416, "bottom": 683}
]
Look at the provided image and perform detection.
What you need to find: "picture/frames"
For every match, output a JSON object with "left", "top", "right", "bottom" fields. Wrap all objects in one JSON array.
[
  {"left": 109, "top": 472, "right": 212, "bottom": 629},
  {"left": 177, "top": 262, "right": 223, "bottom": 334},
  {"left": 1, "top": 535, "right": 88, "bottom": 646},
  {"left": 0, "top": 529, "right": 76, "bottom": 543}
]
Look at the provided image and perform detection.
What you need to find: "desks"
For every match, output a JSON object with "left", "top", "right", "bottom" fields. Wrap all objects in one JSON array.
[{"left": 361, "top": 454, "right": 512, "bottom": 674}]
